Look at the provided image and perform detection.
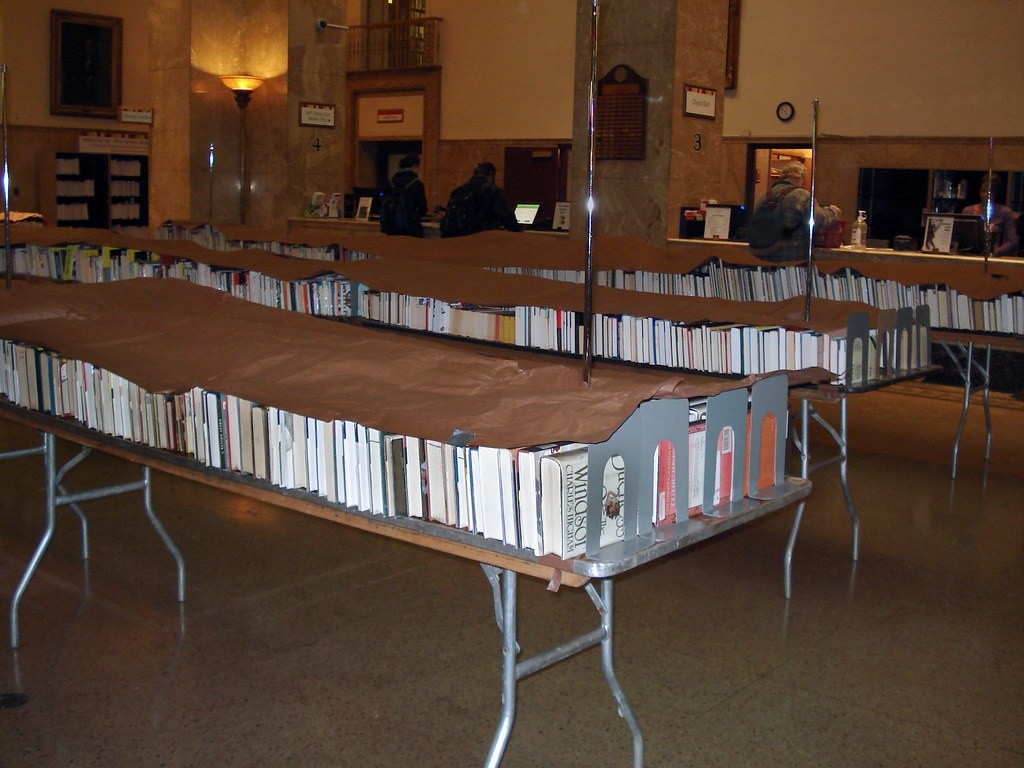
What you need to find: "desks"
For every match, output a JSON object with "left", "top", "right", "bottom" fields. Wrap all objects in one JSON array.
[{"left": 0, "top": 322, "right": 1024, "bottom": 768}]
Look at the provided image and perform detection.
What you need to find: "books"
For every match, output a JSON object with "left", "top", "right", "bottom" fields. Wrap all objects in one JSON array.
[
  {"left": 0, "top": 245, "right": 931, "bottom": 386},
  {"left": 56, "top": 203, "right": 89, "bottom": 220},
  {"left": 110, "top": 160, "right": 141, "bottom": 176},
  {"left": 110, "top": 180, "right": 139, "bottom": 197},
  {"left": 110, "top": 203, "right": 139, "bottom": 220},
  {"left": 56, "top": 180, "right": 95, "bottom": 197},
  {"left": 1, "top": 339, "right": 792, "bottom": 560},
  {"left": 552, "top": 202, "right": 571, "bottom": 230},
  {"left": 703, "top": 207, "right": 731, "bottom": 239},
  {"left": 55, "top": 158, "right": 80, "bottom": 175},
  {"left": 923, "top": 217, "right": 954, "bottom": 254},
  {"left": 356, "top": 197, "right": 373, "bottom": 221},
  {"left": 152, "top": 221, "right": 380, "bottom": 262}
]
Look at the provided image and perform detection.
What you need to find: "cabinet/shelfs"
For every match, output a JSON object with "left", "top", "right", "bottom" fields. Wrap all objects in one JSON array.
[{"left": 54, "top": 151, "right": 150, "bottom": 229}]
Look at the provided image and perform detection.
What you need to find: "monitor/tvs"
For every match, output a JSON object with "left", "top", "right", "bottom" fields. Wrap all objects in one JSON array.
[
  {"left": 511, "top": 202, "right": 540, "bottom": 227},
  {"left": 705, "top": 203, "right": 749, "bottom": 239},
  {"left": 924, "top": 212, "right": 983, "bottom": 254}
]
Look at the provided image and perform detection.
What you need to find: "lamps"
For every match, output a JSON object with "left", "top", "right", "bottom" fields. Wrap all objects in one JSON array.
[{"left": 220, "top": 74, "right": 264, "bottom": 91}]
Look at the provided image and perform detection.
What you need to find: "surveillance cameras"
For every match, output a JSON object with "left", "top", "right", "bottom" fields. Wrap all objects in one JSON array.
[{"left": 317, "top": 17, "right": 330, "bottom": 30}]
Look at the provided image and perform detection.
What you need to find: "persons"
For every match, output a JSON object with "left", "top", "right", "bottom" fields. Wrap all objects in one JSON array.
[
  {"left": 379, "top": 155, "right": 429, "bottom": 238},
  {"left": 926, "top": 218, "right": 937, "bottom": 248},
  {"left": 747, "top": 161, "right": 842, "bottom": 268},
  {"left": 961, "top": 173, "right": 1019, "bottom": 257},
  {"left": 440, "top": 162, "right": 525, "bottom": 238}
]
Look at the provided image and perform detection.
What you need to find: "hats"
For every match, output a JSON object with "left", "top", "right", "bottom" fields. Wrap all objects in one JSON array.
[
  {"left": 474, "top": 162, "right": 494, "bottom": 173},
  {"left": 780, "top": 160, "right": 807, "bottom": 179}
]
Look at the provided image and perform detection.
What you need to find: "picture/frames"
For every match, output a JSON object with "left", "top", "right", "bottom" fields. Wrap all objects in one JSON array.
[{"left": 49, "top": 8, "right": 123, "bottom": 119}]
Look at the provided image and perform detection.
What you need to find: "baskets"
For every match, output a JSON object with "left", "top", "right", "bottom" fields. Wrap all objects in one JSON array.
[{"left": 815, "top": 221, "right": 847, "bottom": 248}]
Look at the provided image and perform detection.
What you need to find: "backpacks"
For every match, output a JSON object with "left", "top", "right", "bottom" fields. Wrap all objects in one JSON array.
[
  {"left": 379, "top": 175, "right": 420, "bottom": 237},
  {"left": 749, "top": 185, "right": 804, "bottom": 256},
  {"left": 441, "top": 196, "right": 482, "bottom": 238}
]
